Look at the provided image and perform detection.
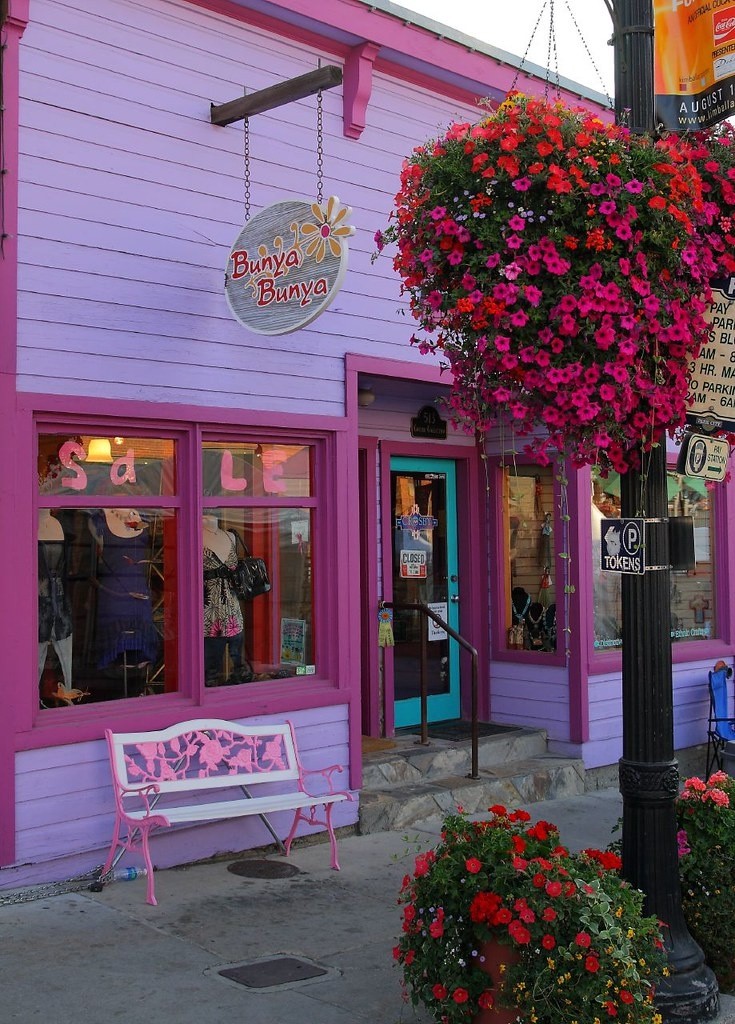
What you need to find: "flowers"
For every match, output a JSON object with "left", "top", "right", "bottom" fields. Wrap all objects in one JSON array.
[
  {"left": 391, "top": 803, "right": 674, "bottom": 1024},
  {"left": 367, "top": 88, "right": 735, "bottom": 480},
  {"left": 608, "top": 770, "right": 735, "bottom": 971}
]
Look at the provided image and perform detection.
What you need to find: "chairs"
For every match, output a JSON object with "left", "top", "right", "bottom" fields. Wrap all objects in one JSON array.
[{"left": 705, "top": 665, "right": 735, "bottom": 783}]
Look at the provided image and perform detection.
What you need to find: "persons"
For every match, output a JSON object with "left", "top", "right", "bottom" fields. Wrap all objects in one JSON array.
[
  {"left": 89, "top": 493, "right": 158, "bottom": 671},
  {"left": 38, "top": 508, "right": 72, "bottom": 691},
  {"left": 202, "top": 515, "right": 244, "bottom": 686}
]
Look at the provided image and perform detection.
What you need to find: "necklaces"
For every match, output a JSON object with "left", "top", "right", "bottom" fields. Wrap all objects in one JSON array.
[
  {"left": 529, "top": 607, "right": 545, "bottom": 623},
  {"left": 112, "top": 508, "right": 134, "bottom": 522},
  {"left": 512, "top": 593, "right": 530, "bottom": 622},
  {"left": 205, "top": 527, "right": 218, "bottom": 535}
]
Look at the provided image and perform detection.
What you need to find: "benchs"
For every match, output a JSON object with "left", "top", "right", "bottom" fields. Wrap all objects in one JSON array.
[{"left": 100, "top": 719, "right": 352, "bottom": 905}]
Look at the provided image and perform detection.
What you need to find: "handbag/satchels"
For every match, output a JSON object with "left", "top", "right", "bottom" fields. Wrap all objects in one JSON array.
[{"left": 226, "top": 528, "right": 271, "bottom": 600}]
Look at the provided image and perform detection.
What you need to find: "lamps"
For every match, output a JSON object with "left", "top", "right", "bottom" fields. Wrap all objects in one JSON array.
[
  {"left": 357, "top": 387, "right": 374, "bottom": 407},
  {"left": 85, "top": 437, "right": 113, "bottom": 462}
]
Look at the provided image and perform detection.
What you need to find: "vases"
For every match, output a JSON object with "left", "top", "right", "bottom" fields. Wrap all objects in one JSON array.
[{"left": 468, "top": 935, "right": 535, "bottom": 1024}]
